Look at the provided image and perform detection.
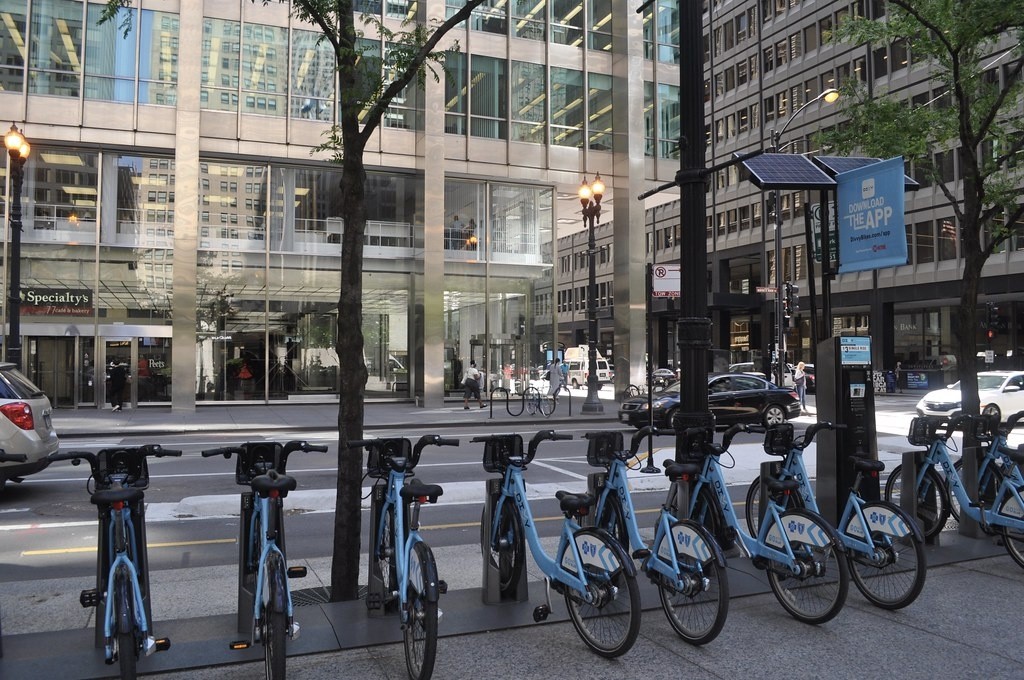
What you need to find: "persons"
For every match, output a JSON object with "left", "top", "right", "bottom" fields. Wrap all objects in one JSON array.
[
  {"left": 447, "top": 215, "right": 486, "bottom": 250},
  {"left": 895, "top": 362, "right": 903, "bottom": 393},
  {"left": 82, "top": 352, "right": 157, "bottom": 412},
  {"left": 463, "top": 360, "right": 489, "bottom": 410},
  {"left": 794, "top": 362, "right": 808, "bottom": 413},
  {"left": 546, "top": 357, "right": 564, "bottom": 405},
  {"left": 559, "top": 359, "right": 571, "bottom": 391}
]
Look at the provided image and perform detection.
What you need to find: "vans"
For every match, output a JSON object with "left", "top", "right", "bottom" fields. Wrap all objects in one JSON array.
[{"left": 562, "top": 359, "right": 611, "bottom": 390}]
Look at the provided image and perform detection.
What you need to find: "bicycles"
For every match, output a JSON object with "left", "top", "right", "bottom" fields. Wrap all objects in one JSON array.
[
  {"left": 526, "top": 382, "right": 556, "bottom": 416},
  {"left": 468, "top": 431, "right": 642, "bottom": 660},
  {"left": 580, "top": 427, "right": 731, "bottom": 647},
  {"left": 883, "top": 409, "right": 1024, "bottom": 571},
  {"left": 48, "top": 443, "right": 182, "bottom": 680},
  {"left": 745, "top": 422, "right": 927, "bottom": 612},
  {"left": 671, "top": 421, "right": 851, "bottom": 625},
  {"left": 345, "top": 437, "right": 460, "bottom": 680},
  {"left": 201, "top": 442, "right": 328, "bottom": 680}
]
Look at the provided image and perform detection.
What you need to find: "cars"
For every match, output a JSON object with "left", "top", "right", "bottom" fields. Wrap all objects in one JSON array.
[
  {"left": 607, "top": 364, "right": 616, "bottom": 384},
  {"left": 728, "top": 361, "right": 797, "bottom": 390},
  {"left": 0, "top": 361, "right": 61, "bottom": 489},
  {"left": 84, "top": 363, "right": 150, "bottom": 396},
  {"left": 508, "top": 363, "right": 549, "bottom": 382},
  {"left": 618, "top": 371, "right": 801, "bottom": 433},
  {"left": 916, "top": 370, "right": 1024, "bottom": 429},
  {"left": 788, "top": 364, "right": 816, "bottom": 388},
  {"left": 645, "top": 369, "right": 678, "bottom": 388}
]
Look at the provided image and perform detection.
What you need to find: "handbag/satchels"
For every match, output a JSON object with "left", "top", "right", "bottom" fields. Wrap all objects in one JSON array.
[
  {"left": 460, "top": 371, "right": 468, "bottom": 385},
  {"left": 545, "top": 371, "right": 550, "bottom": 380},
  {"left": 794, "top": 369, "right": 804, "bottom": 386}
]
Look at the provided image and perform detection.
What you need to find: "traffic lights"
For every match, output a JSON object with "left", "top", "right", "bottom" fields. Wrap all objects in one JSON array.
[
  {"left": 988, "top": 330, "right": 994, "bottom": 338},
  {"left": 986, "top": 303, "right": 999, "bottom": 329},
  {"left": 786, "top": 281, "right": 800, "bottom": 315}
]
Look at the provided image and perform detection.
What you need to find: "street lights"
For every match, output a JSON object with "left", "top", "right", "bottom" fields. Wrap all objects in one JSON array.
[
  {"left": 774, "top": 89, "right": 838, "bottom": 387},
  {"left": 578, "top": 171, "right": 607, "bottom": 415}
]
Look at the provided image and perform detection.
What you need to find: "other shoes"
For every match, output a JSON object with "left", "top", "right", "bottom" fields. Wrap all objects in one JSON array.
[
  {"left": 112, "top": 405, "right": 121, "bottom": 412},
  {"left": 480, "top": 403, "right": 488, "bottom": 408},
  {"left": 464, "top": 406, "right": 470, "bottom": 409}
]
